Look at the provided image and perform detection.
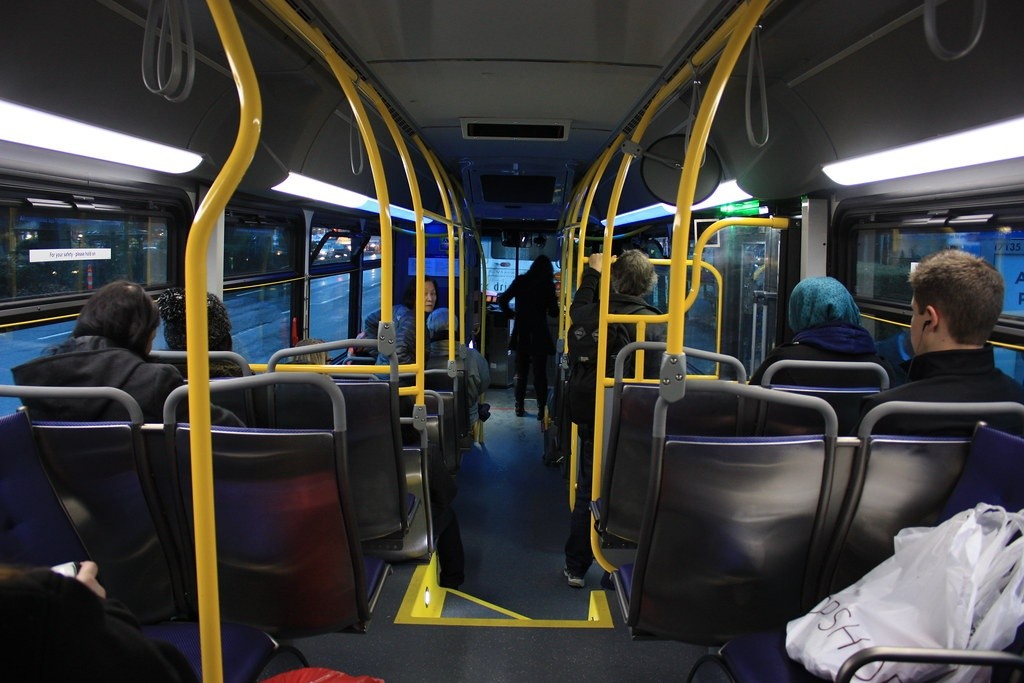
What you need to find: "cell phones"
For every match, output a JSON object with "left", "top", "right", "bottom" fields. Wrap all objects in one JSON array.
[{"left": 50, "top": 562, "right": 80, "bottom": 579}]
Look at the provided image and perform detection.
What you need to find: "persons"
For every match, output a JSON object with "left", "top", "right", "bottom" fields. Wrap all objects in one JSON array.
[
  {"left": 291, "top": 336, "right": 329, "bottom": 365},
  {"left": 853, "top": 248, "right": 1024, "bottom": 434},
  {"left": 499, "top": 254, "right": 560, "bottom": 422},
  {"left": 747, "top": 275, "right": 919, "bottom": 386},
  {"left": 345, "top": 274, "right": 491, "bottom": 434},
  {"left": 10, "top": 279, "right": 278, "bottom": 429},
  {"left": 563, "top": 248, "right": 675, "bottom": 588},
  {"left": 0, "top": 559, "right": 203, "bottom": 683}
]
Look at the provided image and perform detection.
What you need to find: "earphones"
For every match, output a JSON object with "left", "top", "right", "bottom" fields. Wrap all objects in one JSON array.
[{"left": 922, "top": 320, "right": 931, "bottom": 331}]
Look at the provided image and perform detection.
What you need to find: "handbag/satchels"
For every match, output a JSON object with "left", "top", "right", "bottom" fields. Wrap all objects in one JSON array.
[{"left": 786, "top": 503, "right": 1024, "bottom": 683}]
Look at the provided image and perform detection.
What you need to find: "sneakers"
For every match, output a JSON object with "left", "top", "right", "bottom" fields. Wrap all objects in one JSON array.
[{"left": 563, "top": 564, "right": 586, "bottom": 587}]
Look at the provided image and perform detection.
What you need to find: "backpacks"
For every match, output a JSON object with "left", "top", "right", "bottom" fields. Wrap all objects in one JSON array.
[{"left": 568, "top": 304, "right": 646, "bottom": 373}]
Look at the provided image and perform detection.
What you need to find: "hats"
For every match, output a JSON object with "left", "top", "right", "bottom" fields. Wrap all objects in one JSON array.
[{"left": 427, "top": 307, "right": 459, "bottom": 331}]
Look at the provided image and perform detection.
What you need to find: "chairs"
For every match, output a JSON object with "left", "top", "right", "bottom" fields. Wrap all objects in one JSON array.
[{"left": 0, "top": 338, "right": 1024, "bottom": 683}]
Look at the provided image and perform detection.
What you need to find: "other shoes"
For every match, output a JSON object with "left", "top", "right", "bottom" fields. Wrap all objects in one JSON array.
[
  {"left": 536, "top": 408, "right": 544, "bottom": 420},
  {"left": 513, "top": 400, "right": 524, "bottom": 415}
]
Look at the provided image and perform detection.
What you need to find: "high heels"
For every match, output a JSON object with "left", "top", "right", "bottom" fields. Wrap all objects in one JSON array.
[{"left": 478, "top": 403, "right": 491, "bottom": 422}]
